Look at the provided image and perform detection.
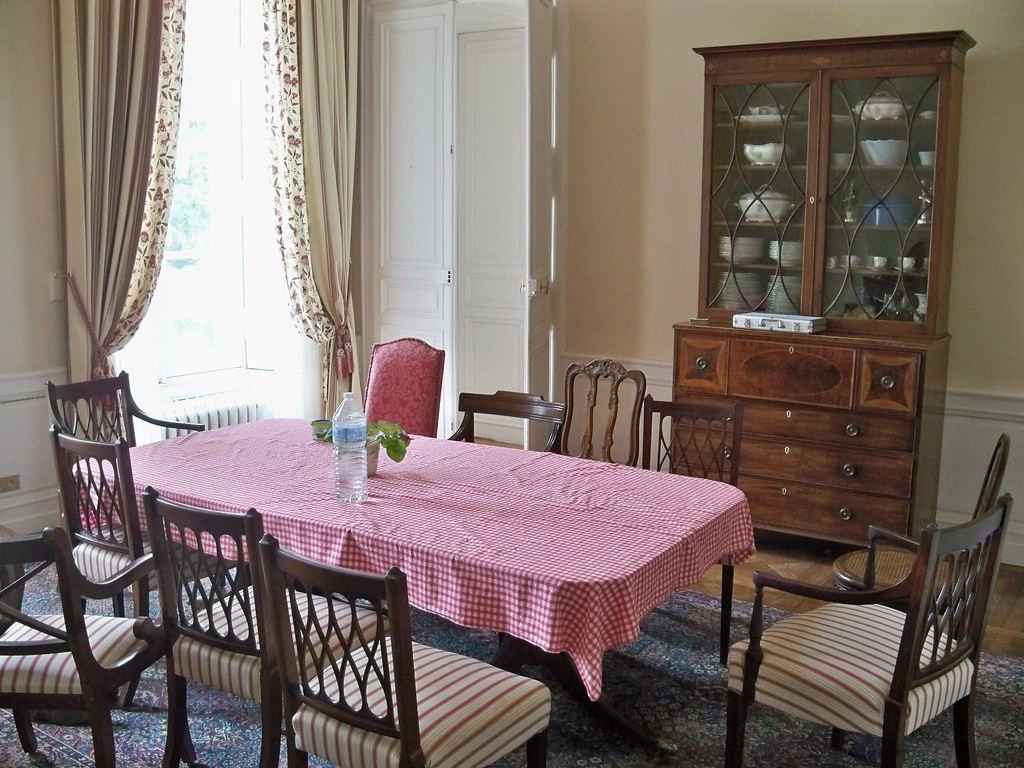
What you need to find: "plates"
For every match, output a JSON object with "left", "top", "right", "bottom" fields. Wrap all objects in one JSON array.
[
  {"left": 839, "top": 264, "right": 861, "bottom": 269},
  {"left": 733, "top": 114, "right": 796, "bottom": 123},
  {"left": 768, "top": 275, "right": 802, "bottom": 315},
  {"left": 769, "top": 241, "right": 803, "bottom": 266},
  {"left": 867, "top": 265, "right": 881, "bottom": 271},
  {"left": 832, "top": 114, "right": 852, "bottom": 123},
  {"left": 716, "top": 272, "right": 768, "bottom": 309},
  {"left": 894, "top": 267, "right": 913, "bottom": 273},
  {"left": 718, "top": 237, "right": 769, "bottom": 263}
]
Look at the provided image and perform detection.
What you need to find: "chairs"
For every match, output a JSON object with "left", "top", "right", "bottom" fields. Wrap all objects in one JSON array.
[
  {"left": 831, "top": 434, "right": 1013, "bottom": 591},
  {"left": 1, "top": 338, "right": 742, "bottom": 768},
  {"left": 725, "top": 493, "right": 1016, "bottom": 768}
]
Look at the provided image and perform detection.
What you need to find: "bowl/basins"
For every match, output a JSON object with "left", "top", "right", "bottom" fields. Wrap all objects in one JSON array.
[
  {"left": 748, "top": 105, "right": 784, "bottom": 114},
  {"left": 919, "top": 111, "right": 935, "bottom": 119},
  {"left": 743, "top": 142, "right": 795, "bottom": 165},
  {"left": 859, "top": 140, "right": 914, "bottom": 167}
]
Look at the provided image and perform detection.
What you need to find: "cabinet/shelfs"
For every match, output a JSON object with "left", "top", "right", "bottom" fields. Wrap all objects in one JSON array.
[{"left": 671, "top": 29, "right": 977, "bottom": 543}]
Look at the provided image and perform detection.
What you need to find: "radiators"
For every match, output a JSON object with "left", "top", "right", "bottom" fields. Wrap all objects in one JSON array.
[{"left": 155, "top": 396, "right": 266, "bottom": 438}]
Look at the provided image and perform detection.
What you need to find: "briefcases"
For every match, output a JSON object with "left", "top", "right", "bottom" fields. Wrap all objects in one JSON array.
[{"left": 733, "top": 312, "right": 826, "bottom": 333}]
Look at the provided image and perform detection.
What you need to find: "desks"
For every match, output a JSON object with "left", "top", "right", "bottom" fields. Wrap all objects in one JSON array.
[{"left": 70, "top": 415, "right": 757, "bottom": 768}]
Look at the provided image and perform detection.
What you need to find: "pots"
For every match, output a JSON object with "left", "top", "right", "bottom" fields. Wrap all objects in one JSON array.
[
  {"left": 734, "top": 184, "right": 792, "bottom": 223},
  {"left": 857, "top": 197, "right": 915, "bottom": 225},
  {"left": 852, "top": 91, "right": 909, "bottom": 121}
]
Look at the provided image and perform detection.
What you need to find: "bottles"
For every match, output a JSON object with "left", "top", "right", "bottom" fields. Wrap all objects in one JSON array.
[
  {"left": 332, "top": 392, "right": 368, "bottom": 504},
  {"left": 913, "top": 293, "right": 927, "bottom": 324}
]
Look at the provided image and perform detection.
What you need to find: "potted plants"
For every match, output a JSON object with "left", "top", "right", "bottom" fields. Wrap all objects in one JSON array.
[{"left": 311, "top": 419, "right": 413, "bottom": 478}]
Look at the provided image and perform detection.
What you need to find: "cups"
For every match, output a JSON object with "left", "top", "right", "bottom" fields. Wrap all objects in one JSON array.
[
  {"left": 841, "top": 255, "right": 861, "bottom": 266},
  {"left": 826, "top": 256, "right": 837, "bottom": 270},
  {"left": 924, "top": 258, "right": 929, "bottom": 262},
  {"left": 867, "top": 256, "right": 887, "bottom": 267},
  {"left": 897, "top": 256, "right": 915, "bottom": 268},
  {"left": 312, "top": 419, "right": 331, "bottom": 444},
  {"left": 835, "top": 153, "right": 852, "bottom": 168},
  {"left": 919, "top": 151, "right": 933, "bottom": 166}
]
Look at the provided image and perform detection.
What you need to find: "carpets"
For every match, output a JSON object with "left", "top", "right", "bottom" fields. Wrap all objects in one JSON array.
[{"left": 1, "top": 532, "right": 1024, "bottom": 768}]
